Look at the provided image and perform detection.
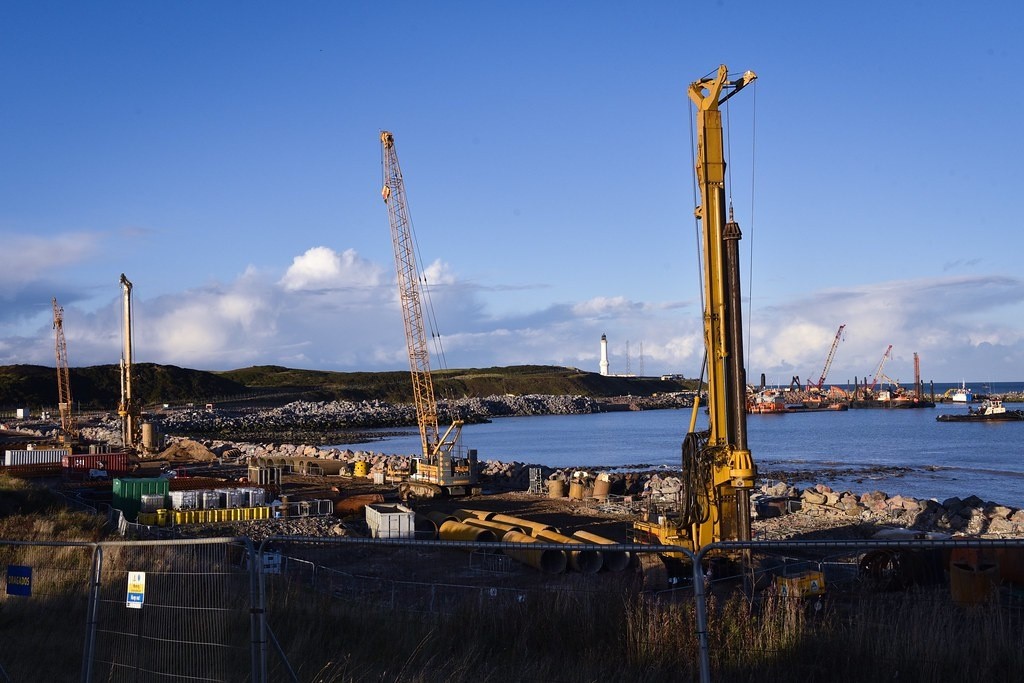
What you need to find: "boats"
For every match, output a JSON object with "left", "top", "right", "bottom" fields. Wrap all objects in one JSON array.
[
  {"left": 740, "top": 323, "right": 849, "bottom": 415},
  {"left": 935, "top": 397, "right": 1024, "bottom": 423},
  {"left": 844, "top": 345, "right": 936, "bottom": 409}
]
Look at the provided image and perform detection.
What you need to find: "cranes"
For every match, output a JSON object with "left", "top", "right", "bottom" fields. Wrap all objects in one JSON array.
[
  {"left": 810, "top": 322, "right": 846, "bottom": 391},
  {"left": 116, "top": 270, "right": 136, "bottom": 461},
  {"left": 866, "top": 344, "right": 900, "bottom": 395},
  {"left": 431, "top": 63, "right": 827, "bottom": 631},
  {"left": 372, "top": 126, "right": 478, "bottom": 511},
  {"left": 49, "top": 295, "right": 81, "bottom": 445}
]
